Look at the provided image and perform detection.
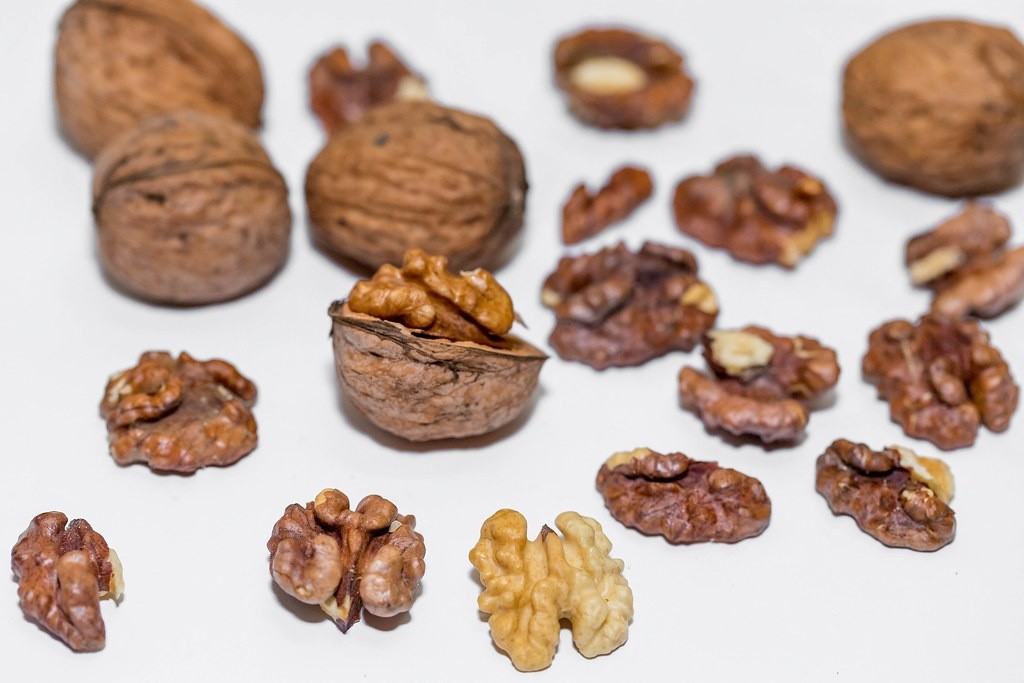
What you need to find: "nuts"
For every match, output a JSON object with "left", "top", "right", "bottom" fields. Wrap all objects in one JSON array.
[{"left": 12, "top": 0, "right": 1024, "bottom": 671}]
